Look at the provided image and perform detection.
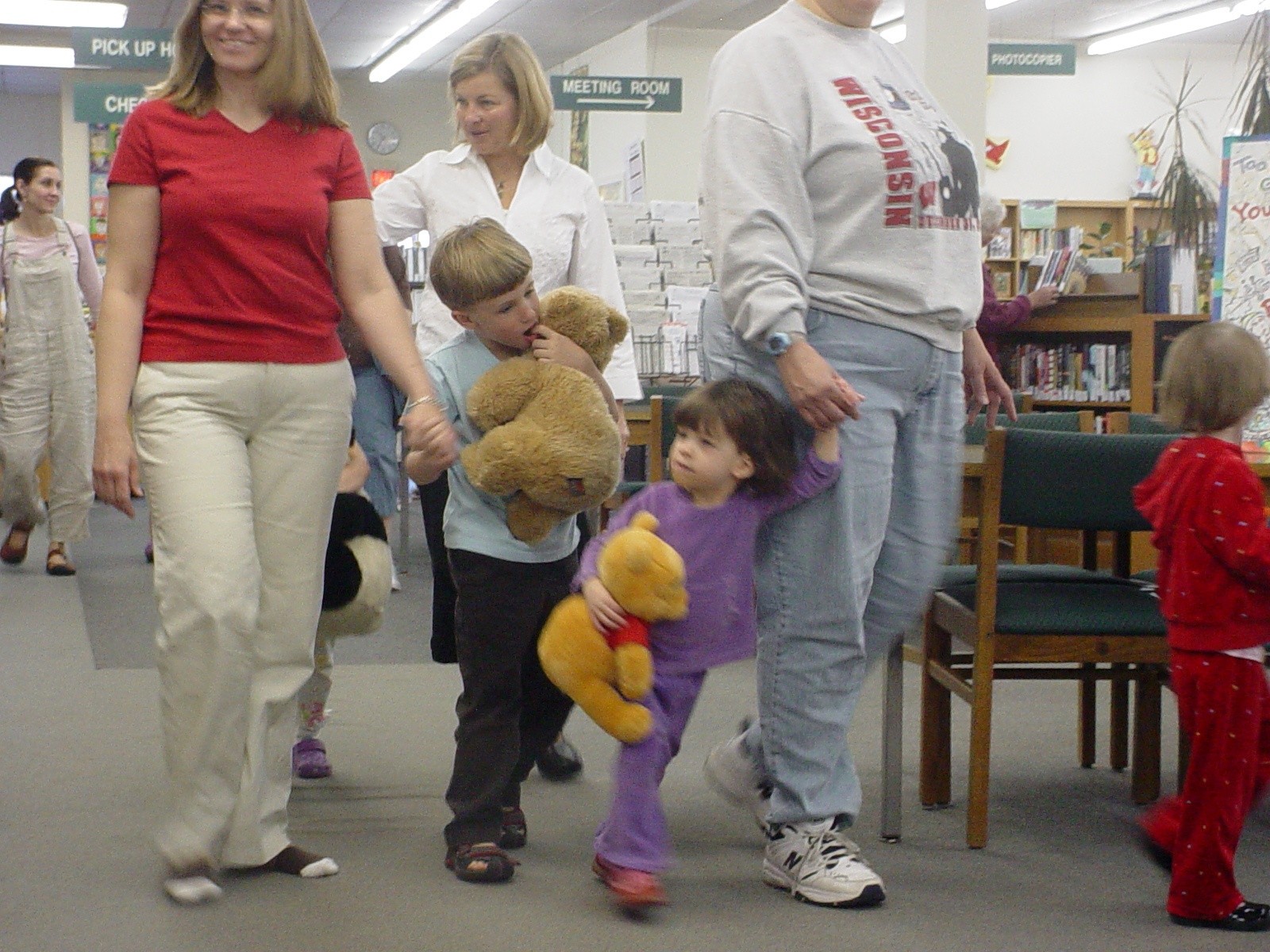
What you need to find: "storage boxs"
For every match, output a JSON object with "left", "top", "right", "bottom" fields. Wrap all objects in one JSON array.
[{"left": 1029, "top": 267, "right": 1144, "bottom": 317}]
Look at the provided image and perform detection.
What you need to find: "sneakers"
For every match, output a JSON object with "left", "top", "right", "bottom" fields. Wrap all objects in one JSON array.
[
  {"left": 390, "top": 563, "right": 401, "bottom": 590},
  {"left": 762, "top": 827, "right": 888, "bottom": 908},
  {"left": 704, "top": 733, "right": 773, "bottom": 834}
]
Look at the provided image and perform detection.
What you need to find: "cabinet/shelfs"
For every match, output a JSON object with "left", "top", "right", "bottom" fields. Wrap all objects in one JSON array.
[
  {"left": 983, "top": 198, "right": 1218, "bottom": 307},
  {"left": 1003, "top": 313, "right": 1208, "bottom": 415}
]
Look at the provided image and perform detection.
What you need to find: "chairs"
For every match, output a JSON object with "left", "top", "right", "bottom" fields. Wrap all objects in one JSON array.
[{"left": 618, "top": 393, "right": 1191, "bottom": 858}]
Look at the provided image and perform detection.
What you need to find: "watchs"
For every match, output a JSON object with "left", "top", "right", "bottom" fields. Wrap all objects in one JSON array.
[{"left": 764, "top": 329, "right": 806, "bottom": 359}]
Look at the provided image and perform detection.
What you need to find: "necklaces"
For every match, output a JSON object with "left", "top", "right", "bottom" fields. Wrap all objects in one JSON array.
[{"left": 484, "top": 164, "right": 523, "bottom": 197}]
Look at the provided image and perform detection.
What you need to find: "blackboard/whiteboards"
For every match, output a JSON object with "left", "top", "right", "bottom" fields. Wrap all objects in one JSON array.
[{"left": 1210, "top": 134, "right": 1270, "bottom": 453}]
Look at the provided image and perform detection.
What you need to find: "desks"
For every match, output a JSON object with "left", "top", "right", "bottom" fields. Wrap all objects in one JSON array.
[{"left": 880, "top": 438, "right": 1270, "bottom": 849}]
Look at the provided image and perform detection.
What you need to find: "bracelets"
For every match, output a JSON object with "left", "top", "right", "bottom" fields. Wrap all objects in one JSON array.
[{"left": 404, "top": 394, "right": 440, "bottom": 407}]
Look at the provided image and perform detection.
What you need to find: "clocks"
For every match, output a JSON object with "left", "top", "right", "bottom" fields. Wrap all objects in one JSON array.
[{"left": 366, "top": 121, "right": 402, "bottom": 156}]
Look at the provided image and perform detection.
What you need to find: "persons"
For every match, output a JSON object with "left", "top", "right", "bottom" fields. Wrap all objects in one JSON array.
[
  {"left": 289, "top": 233, "right": 414, "bottom": 780},
  {"left": 695, "top": 0, "right": 1018, "bottom": 910},
  {"left": 970, "top": 186, "right": 1059, "bottom": 398},
  {"left": 1132, "top": 320, "right": 1269, "bottom": 934},
  {"left": 0, "top": 157, "right": 102, "bottom": 577},
  {"left": 402, "top": 217, "right": 633, "bottom": 885},
  {"left": 88, "top": 0, "right": 452, "bottom": 907},
  {"left": 368, "top": 31, "right": 644, "bottom": 782},
  {"left": 565, "top": 377, "right": 844, "bottom": 906}
]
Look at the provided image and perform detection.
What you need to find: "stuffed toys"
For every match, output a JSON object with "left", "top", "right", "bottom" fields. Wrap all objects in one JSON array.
[
  {"left": 458, "top": 282, "right": 633, "bottom": 543},
  {"left": 536, "top": 509, "right": 693, "bottom": 744}
]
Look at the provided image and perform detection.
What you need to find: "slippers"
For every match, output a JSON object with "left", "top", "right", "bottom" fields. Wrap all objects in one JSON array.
[{"left": 292, "top": 739, "right": 330, "bottom": 779}]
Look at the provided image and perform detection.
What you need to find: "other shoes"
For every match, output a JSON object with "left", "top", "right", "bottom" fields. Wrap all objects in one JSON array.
[
  {"left": 45, "top": 549, "right": 76, "bottom": 575},
  {"left": 0, "top": 523, "right": 30, "bottom": 564},
  {"left": 1129, "top": 822, "right": 1173, "bottom": 874},
  {"left": 536, "top": 732, "right": 582, "bottom": 781},
  {"left": 1171, "top": 900, "right": 1269, "bottom": 931},
  {"left": 593, "top": 852, "right": 670, "bottom": 905}
]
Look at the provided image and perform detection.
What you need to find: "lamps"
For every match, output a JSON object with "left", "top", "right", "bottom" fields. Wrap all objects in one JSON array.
[
  {"left": 369, "top": 0, "right": 498, "bottom": 83},
  {"left": 1083, "top": 0, "right": 1270, "bottom": 58}
]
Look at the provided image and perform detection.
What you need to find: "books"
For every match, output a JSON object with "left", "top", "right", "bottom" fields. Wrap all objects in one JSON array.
[{"left": 982, "top": 225, "right": 1136, "bottom": 404}]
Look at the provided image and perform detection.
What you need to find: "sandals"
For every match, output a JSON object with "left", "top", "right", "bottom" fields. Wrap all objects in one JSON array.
[
  {"left": 497, "top": 805, "right": 528, "bottom": 850},
  {"left": 445, "top": 842, "right": 515, "bottom": 883}
]
